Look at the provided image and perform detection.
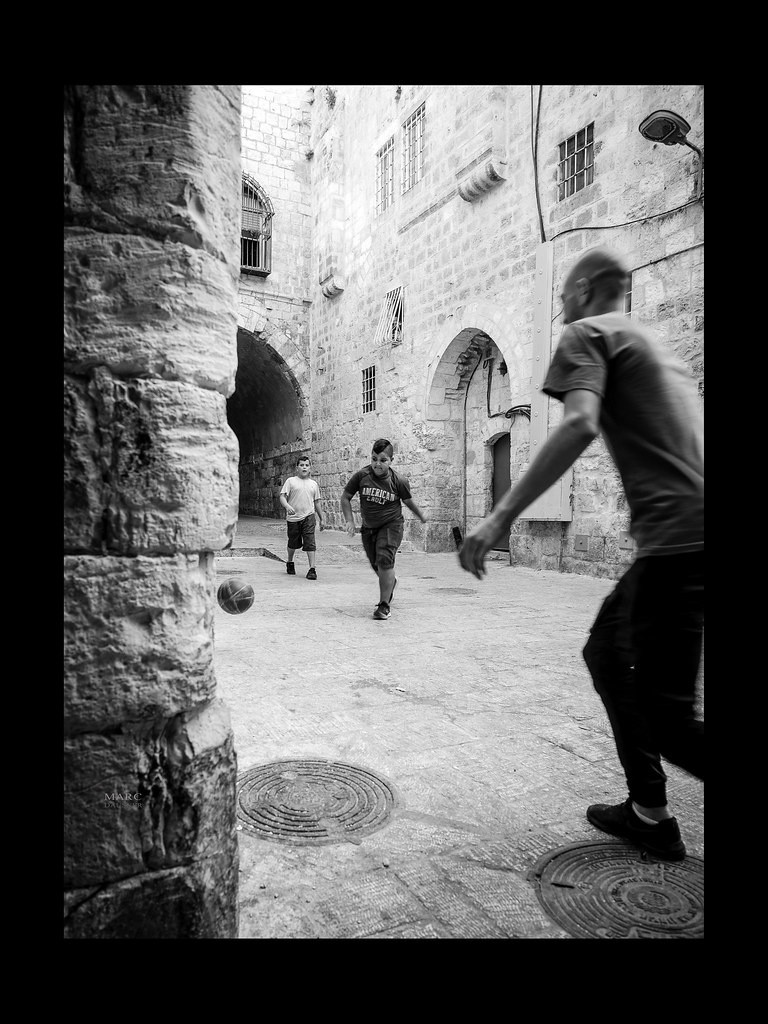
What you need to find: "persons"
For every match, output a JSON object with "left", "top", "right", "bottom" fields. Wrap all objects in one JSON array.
[
  {"left": 339, "top": 438, "right": 427, "bottom": 621},
  {"left": 453, "top": 244, "right": 706, "bottom": 867},
  {"left": 279, "top": 456, "right": 326, "bottom": 580}
]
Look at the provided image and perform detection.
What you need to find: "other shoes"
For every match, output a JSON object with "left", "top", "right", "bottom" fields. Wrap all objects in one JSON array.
[
  {"left": 587, "top": 797, "right": 686, "bottom": 862},
  {"left": 286, "top": 562, "right": 295, "bottom": 574},
  {"left": 373, "top": 601, "right": 391, "bottom": 620},
  {"left": 306, "top": 568, "right": 317, "bottom": 580}
]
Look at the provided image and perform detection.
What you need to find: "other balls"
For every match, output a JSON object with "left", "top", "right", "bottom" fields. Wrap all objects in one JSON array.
[{"left": 218, "top": 576, "right": 254, "bottom": 614}]
